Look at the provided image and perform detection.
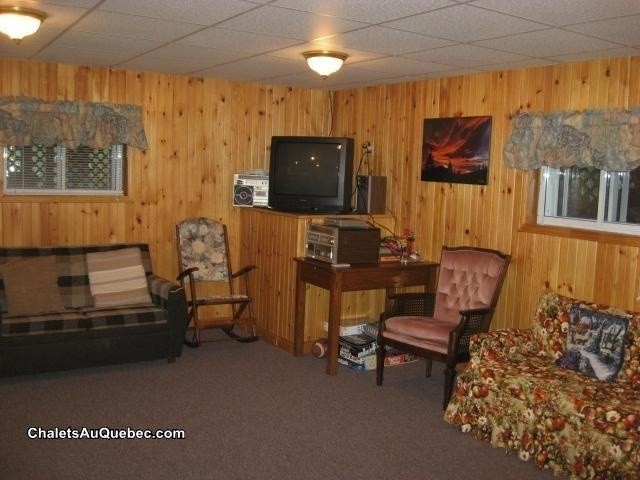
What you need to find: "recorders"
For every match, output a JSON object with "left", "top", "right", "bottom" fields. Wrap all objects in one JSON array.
[{"left": 232, "top": 174, "right": 269, "bottom": 208}]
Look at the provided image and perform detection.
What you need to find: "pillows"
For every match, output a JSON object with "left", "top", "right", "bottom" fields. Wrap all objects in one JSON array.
[
  {"left": 1, "top": 254, "right": 67, "bottom": 317},
  {"left": 553, "top": 302, "right": 629, "bottom": 387},
  {"left": 82, "top": 247, "right": 154, "bottom": 309}
]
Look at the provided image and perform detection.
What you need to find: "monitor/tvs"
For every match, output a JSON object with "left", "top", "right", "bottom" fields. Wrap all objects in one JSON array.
[{"left": 269, "top": 136, "right": 354, "bottom": 214}]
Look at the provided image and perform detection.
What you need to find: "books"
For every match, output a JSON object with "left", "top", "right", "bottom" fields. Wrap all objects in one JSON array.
[{"left": 323, "top": 318, "right": 420, "bottom": 371}]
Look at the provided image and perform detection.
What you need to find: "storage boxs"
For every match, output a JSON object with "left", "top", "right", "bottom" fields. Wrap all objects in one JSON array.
[
  {"left": 322, "top": 316, "right": 376, "bottom": 336},
  {"left": 338, "top": 354, "right": 378, "bottom": 371},
  {"left": 383, "top": 349, "right": 424, "bottom": 368},
  {"left": 338, "top": 336, "right": 394, "bottom": 359}
]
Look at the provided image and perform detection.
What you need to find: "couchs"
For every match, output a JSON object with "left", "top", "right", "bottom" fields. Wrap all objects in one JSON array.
[
  {"left": 440, "top": 290, "right": 640, "bottom": 480},
  {"left": 0, "top": 241, "right": 186, "bottom": 381}
]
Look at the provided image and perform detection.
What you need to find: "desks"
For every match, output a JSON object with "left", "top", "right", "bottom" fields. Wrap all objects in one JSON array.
[{"left": 291, "top": 254, "right": 441, "bottom": 375}]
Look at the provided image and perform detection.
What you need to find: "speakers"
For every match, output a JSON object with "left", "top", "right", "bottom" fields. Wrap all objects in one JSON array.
[{"left": 357, "top": 175, "right": 387, "bottom": 214}]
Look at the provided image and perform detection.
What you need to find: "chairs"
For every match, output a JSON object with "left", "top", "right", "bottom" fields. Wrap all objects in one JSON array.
[
  {"left": 373, "top": 242, "right": 513, "bottom": 412},
  {"left": 173, "top": 217, "right": 262, "bottom": 350}
]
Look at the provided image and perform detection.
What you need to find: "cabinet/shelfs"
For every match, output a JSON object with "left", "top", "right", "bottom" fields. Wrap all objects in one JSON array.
[{"left": 239, "top": 208, "right": 397, "bottom": 359}]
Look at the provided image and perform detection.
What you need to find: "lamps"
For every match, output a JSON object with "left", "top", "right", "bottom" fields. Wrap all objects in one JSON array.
[
  {"left": 0, "top": 6, "right": 47, "bottom": 42},
  {"left": 302, "top": 50, "right": 351, "bottom": 78}
]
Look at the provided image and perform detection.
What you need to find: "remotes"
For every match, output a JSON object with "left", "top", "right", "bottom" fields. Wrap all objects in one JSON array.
[{"left": 331, "top": 263, "right": 351, "bottom": 267}]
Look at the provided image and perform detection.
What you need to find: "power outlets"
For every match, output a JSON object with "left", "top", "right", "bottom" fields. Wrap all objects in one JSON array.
[{"left": 365, "top": 141, "right": 374, "bottom": 154}]
